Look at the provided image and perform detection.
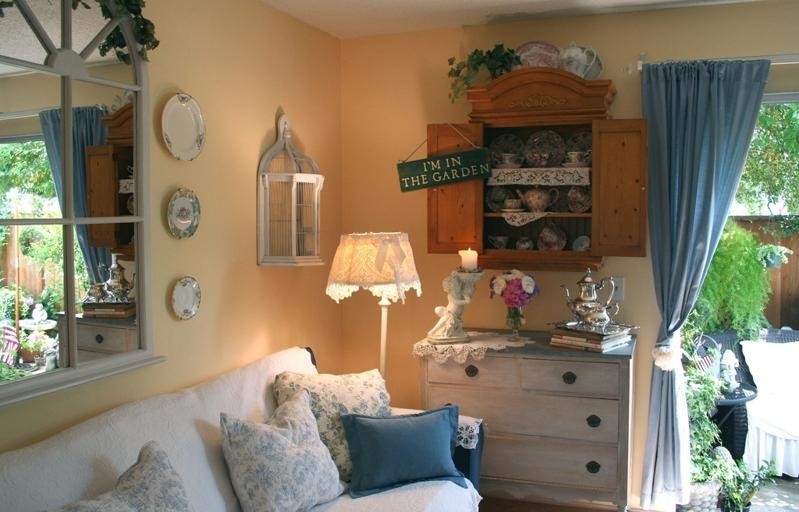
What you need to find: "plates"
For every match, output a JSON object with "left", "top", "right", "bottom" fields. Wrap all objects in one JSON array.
[
  {"left": 168, "top": 187, "right": 201, "bottom": 241},
  {"left": 565, "top": 131, "right": 592, "bottom": 167},
  {"left": 526, "top": 130, "right": 565, "bottom": 166},
  {"left": 512, "top": 42, "right": 560, "bottom": 72},
  {"left": 567, "top": 183, "right": 592, "bottom": 213},
  {"left": 500, "top": 208, "right": 526, "bottom": 212},
  {"left": 561, "top": 163, "right": 590, "bottom": 168},
  {"left": 486, "top": 186, "right": 520, "bottom": 211},
  {"left": 488, "top": 134, "right": 525, "bottom": 167},
  {"left": 171, "top": 277, "right": 202, "bottom": 320},
  {"left": 536, "top": 229, "right": 567, "bottom": 252},
  {"left": 159, "top": 92, "right": 206, "bottom": 162}
]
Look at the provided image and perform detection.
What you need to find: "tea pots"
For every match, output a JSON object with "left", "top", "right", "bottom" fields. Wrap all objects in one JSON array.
[
  {"left": 85, "top": 283, "right": 107, "bottom": 302},
  {"left": 558, "top": 42, "right": 597, "bottom": 79},
  {"left": 514, "top": 184, "right": 560, "bottom": 213},
  {"left": 560, "top": 266, "right": 615, "bottom": 327},
  {"left": 574, "top": 304, "right": 618, "bottom": 330},
  {"left": 97, "top": 262, "right": 135, "bottom": 303}
]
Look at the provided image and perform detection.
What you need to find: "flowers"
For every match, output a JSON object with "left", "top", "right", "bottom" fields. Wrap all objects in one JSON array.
[{"left": 484, "top": 266, "right": 542, "bottom": 327}]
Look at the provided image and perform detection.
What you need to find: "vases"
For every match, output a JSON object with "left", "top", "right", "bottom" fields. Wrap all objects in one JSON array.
[{"left": 505, "top": 306, "right": 526, "bottom": 329}]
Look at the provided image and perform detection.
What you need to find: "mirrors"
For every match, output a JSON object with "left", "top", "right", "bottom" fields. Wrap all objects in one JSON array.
[{"left": 0, "top": 0, "right": 166, "bottom": 407}]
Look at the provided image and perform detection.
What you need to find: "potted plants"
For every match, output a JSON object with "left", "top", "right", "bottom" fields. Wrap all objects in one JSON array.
[
  {"left": 439, "top": 39, "right": 522, "bottom": 104},
  {"left": 714, "top": 458, "right": 775, "bottom": 512},
  {"left": 752, "top": 242, "right": 795, "bottom": 269},
  {"left": 19, "top": 335, "right": 44, "bottom": 363}
]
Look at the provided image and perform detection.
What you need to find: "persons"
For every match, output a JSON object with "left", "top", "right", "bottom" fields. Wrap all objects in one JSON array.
[{"left": 424, "top": 274, "right": 477, "bottom": 344}]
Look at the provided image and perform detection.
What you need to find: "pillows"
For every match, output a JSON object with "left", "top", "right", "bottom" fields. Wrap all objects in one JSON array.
[
  {"left": 68, "top": 436, "right": 194, "bottom": 512},
  {"left": 214, "top": 385, "right": 353, "bottom": 512},
  {"left": 738, "top": 340, "right": 798, "bottom": 394},
  {"left": 340, "top": 400, "right": 467, "bottom": 500},
  {"left": 272, "top": 364, "right": 395, "bottom": 487}
]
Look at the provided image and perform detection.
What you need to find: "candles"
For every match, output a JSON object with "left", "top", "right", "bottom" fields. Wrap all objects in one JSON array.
[{"left": 456, "top": 245, "right": 479, "bottom": 271}]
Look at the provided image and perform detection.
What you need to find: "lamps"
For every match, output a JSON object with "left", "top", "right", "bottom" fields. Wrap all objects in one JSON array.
[{"left": 324, "top": 229, "right": 424, "bottom": 382}]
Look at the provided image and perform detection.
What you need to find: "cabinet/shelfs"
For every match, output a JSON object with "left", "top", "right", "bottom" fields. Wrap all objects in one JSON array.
[
  {"left": 56, "top": 308, "right": 139, "bottom": 369},
  {"left": 419, "top": 68, "right": 654, "bottom": 273},
  {"left": 419, "top": 329, "right": 640, "bottom": 512},
  {"left": 81, "top": 98, "right": 136, "bottom": 261}
]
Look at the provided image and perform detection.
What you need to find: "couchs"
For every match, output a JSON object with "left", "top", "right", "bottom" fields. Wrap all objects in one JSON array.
[{"left": 0, "top": 343, "right": 486, "bottom": 512}]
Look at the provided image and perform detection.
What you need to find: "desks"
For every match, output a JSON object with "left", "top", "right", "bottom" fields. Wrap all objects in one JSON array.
[
  {"left": 712, "top": 380, "right": 756, "bottom": 431},
  {"left": 19, "top": 319, "right": 58, "bottom": 336}
]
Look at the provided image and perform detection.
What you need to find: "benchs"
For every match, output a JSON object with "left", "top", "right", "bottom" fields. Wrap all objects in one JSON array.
[{"left": 701, "top": 328, "right": 798, "bottom": 481}]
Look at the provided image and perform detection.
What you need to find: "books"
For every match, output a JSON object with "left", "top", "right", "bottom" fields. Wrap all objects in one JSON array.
[
  {"left": 81, "top": 294, "right": 137, "bottom": 321},
  {"left": 547, "top": 319, "right": 635, "bottom": 356}
]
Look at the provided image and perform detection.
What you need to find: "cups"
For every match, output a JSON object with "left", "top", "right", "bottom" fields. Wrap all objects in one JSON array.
[
  {"left": 568, "top": 151, "right": 589, "bottom": 163},
  {"left": 572, "top": 236, "right": 591, "bottom": 252},
  {"left": 505, "top": 199, "right": 521, "bottom": 209},
  {"left": 488, "top": 234, "right": 510, "bottom": 248},
  {"left": 493, "top": 153, "right": 519, "bottom": 166},
  {"left": 527, "top": 153, "right": 548, "bottom": 168}
]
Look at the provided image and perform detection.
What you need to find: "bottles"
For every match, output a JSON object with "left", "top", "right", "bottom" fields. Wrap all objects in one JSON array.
[{"left": 515, "top": 236, "right": 534, "bottom": 250}]
